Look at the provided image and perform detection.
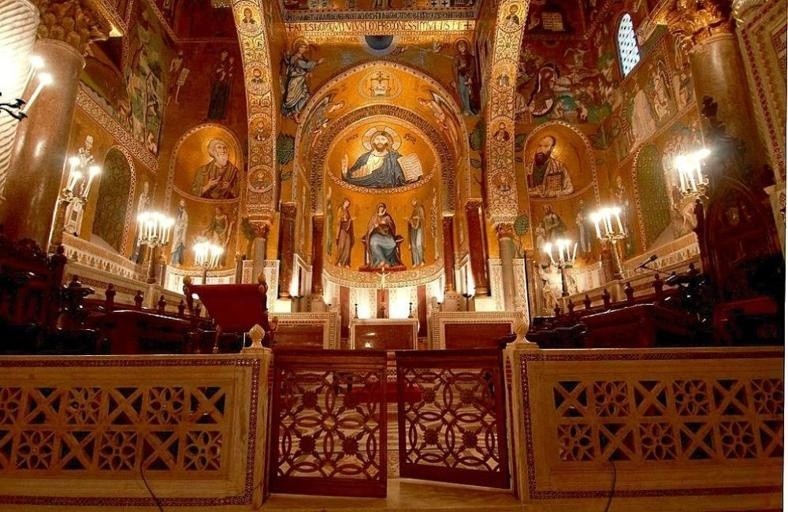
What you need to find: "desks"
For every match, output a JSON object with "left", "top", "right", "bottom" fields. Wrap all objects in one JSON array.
[
  {"left": 268, "top": 313, "right": 342, "bottom": 349},
  {"left": 349, "top": 318, "right": 419, "bottom": 350},
  {"left": 430, "top": 310, "right": 518, "bottom": 350}
]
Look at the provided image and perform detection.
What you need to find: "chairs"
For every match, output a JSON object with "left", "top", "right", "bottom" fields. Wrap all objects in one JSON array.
[
  {"left": 0, "top": 229, "right": 106, "bottom": 351},
  {"left": 292, "top": 292, "right": 340, "bottom": 312},
  {"left": 180, "top": 275, "right": 271, "bottom": 352},
  {"left": 429, "top": 290, "right": 476, "bottom": 311},
  {"left": 677, "top": 171, "right": 785, "bottom": 345}
]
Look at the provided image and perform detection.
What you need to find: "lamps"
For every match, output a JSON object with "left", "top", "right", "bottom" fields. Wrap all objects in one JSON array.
[
  {"left": 192, "top": 241, "right": 224, "bottom": 267},
  {"left": 62, "top": 156, "right": 101, "bottom": 204},
  {"left": 0, "top": 53, "right": 50, "bottom": 120},
  {"left": 136, "top": 210, "right": 174, "bottom": 247}
]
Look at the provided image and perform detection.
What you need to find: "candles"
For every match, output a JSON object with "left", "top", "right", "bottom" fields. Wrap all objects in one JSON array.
[{"left": 542, "top": 151, "right": 706, "bottom": 264}]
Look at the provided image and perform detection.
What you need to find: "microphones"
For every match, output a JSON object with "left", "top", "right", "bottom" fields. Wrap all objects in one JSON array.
[{"left": 634, "top": 254, "right": 658, "bottom": 274}]
